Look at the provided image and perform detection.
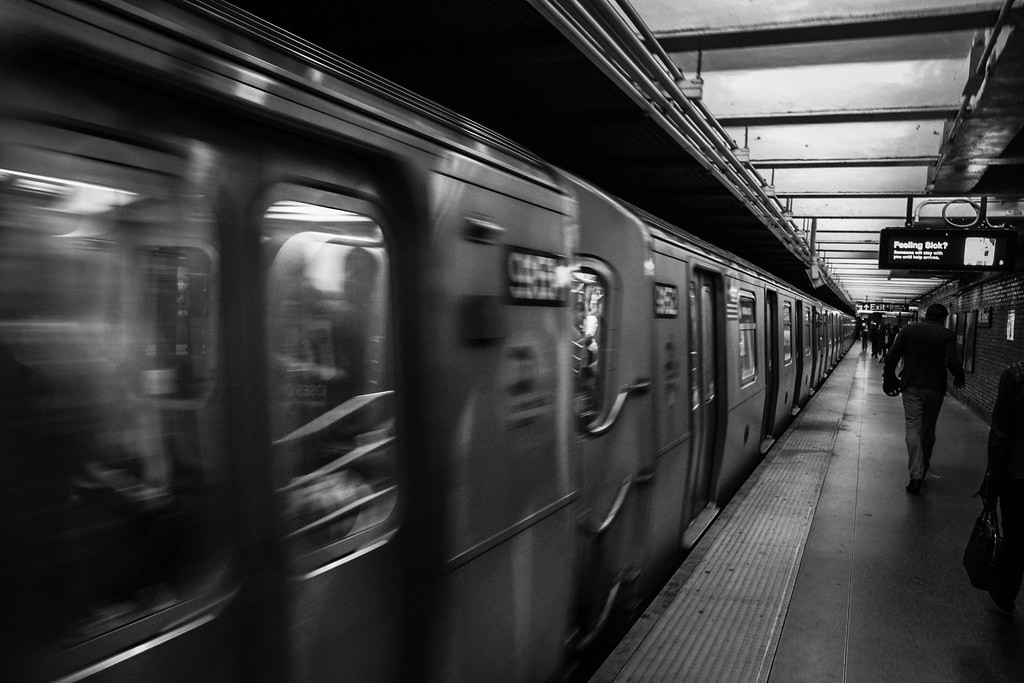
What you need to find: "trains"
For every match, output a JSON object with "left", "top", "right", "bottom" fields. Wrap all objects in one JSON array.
[{"left": 0, "top": 0, "right": 858, "bottom": 683}]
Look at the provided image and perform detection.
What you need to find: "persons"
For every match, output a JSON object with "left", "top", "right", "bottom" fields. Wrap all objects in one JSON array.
[
  {"left": 882, "top": 304, "right": 967, "bottom": 496},
  {"left": 974, "top": 362, "right": 1024, "bottom": 613},
  {"left": 270, "top": 238, "right": 402, "bottom": 508},
  {"left": 862, "top": 320, "right": 901, "bottom": 366}
]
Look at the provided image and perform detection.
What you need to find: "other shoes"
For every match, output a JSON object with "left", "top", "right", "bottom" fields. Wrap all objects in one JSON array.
[
  {"left": 872, "top": 353, "right": 878, "bottom": 358},
  {"left": 905, "top": 480, "right": 922, "bottom": 494},
  {"left": 987, "top": 590, "right": 1018, "bottom": 615}
]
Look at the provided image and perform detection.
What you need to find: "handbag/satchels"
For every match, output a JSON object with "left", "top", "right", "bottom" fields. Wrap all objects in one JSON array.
[{"left": 962, "top": 507, "right": 1006, "bottom": 591}]
